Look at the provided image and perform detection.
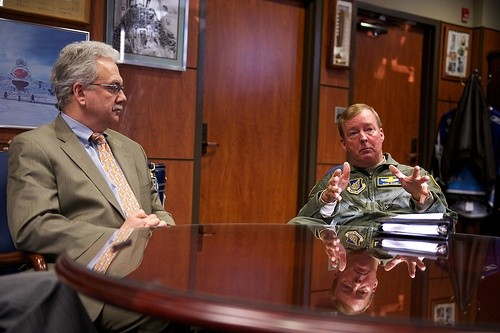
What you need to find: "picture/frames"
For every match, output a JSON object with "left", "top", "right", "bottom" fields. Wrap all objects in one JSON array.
[
  {"left": 442, "top": 24, "right": 473, "bottom": 81},
  {"left": 104, "top": 0, "right": 189, "bottom": 76},
  {"left": 0, "top": 19, "right": 91, "bottom": 129},
  {"left": 326, "top": 0, "right": 355, "bottom": 71}
]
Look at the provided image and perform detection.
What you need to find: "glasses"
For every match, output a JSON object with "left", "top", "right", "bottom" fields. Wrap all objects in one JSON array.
[{"left": 70, "top": 81, "right": 126, "bottom": 95}]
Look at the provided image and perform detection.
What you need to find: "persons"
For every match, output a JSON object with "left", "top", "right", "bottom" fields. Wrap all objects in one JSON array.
[
  {"left": 0, "top": 274, "right": 98, "bottom": 333},
  {"left": 7, "top": 41, "right": 177, "bottom": 333},
  {"left": 7, "top": 58, "right": 32, "bottom": 90},
  {"left": 308, "top": 225, "right": 426, "bottom": 315},
  {"left": 288, "top": 103, "right": 457, "bottom": 225}
]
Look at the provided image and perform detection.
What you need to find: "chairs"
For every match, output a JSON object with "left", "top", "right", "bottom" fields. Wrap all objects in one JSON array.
[{"left": 0, "top": 250, "right": 47, "bottom": 272}]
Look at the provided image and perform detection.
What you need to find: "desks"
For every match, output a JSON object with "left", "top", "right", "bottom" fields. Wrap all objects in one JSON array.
[{"left": 46, "top": 219, "right": 500, "bottom": 333}]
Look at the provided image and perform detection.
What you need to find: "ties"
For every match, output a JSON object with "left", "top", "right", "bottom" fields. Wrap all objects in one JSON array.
[
  {"left": 93, "top": 228, "right": 134, "bottom": 275},
  {"left": 91, "top": 132, "right": 140, "bottom": 219}
]
